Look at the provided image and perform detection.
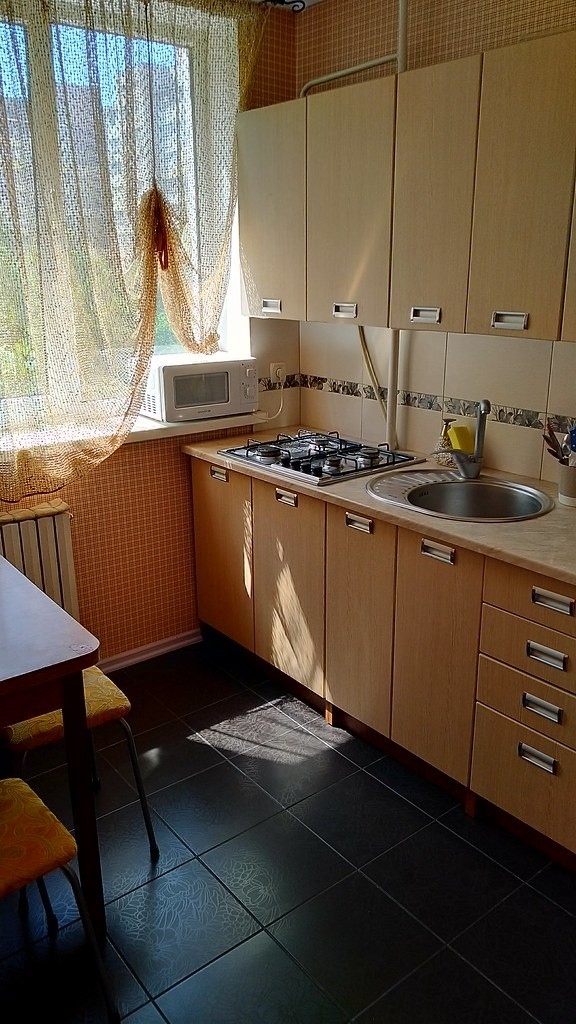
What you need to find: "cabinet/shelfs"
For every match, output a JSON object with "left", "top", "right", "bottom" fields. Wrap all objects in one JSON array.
[
  {"left": 234, "top": 28, "right": 576, "bottom": 343},
  {"left": 187, "top": 423, "right": 576, "bottom": 869}
]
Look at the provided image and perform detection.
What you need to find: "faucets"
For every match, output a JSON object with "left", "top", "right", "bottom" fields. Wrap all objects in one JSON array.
[{"left": 430, "top": 399, "right": 491, "bottom": 479}]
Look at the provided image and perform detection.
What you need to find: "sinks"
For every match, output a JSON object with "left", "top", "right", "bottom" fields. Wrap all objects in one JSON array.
[{"left": 407, "top": 482, "right": 542, "bottom": 518}]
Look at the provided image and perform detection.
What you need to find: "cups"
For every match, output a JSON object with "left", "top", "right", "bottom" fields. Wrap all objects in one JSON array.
[{"left": 559, "top": 459, "right": 576, "bottom": 507}]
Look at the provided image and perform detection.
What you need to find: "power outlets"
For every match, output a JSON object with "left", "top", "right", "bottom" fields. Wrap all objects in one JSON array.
[{"left": 270, "top": 362, "right": 287, "bottom": 383}]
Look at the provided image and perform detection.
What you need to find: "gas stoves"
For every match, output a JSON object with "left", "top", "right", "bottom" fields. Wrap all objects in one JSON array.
[{"left": 217, "top": 429, "right": 426, "bottom": 485}]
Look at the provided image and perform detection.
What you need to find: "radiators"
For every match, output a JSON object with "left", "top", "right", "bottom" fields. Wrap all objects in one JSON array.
[{"left": 0, "top": 497, "right": 80, "bottom": 622}]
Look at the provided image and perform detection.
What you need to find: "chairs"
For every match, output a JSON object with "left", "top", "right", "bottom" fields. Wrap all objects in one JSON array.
[
  {"left": 0, "top": 777, "right": 121, "bottom": 1024},
  {"left": 0, "top": 663, "right": 159, "bottom": 920}
]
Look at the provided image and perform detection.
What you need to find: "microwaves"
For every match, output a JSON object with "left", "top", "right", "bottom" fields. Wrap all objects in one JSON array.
[{"left": 129, "top": 355, "right": 259, "bottom": 423}]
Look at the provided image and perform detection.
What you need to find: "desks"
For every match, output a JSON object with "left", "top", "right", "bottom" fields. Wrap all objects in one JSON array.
[{"left": 0, "top": 551, "right": 109, "bottom": 953}]
